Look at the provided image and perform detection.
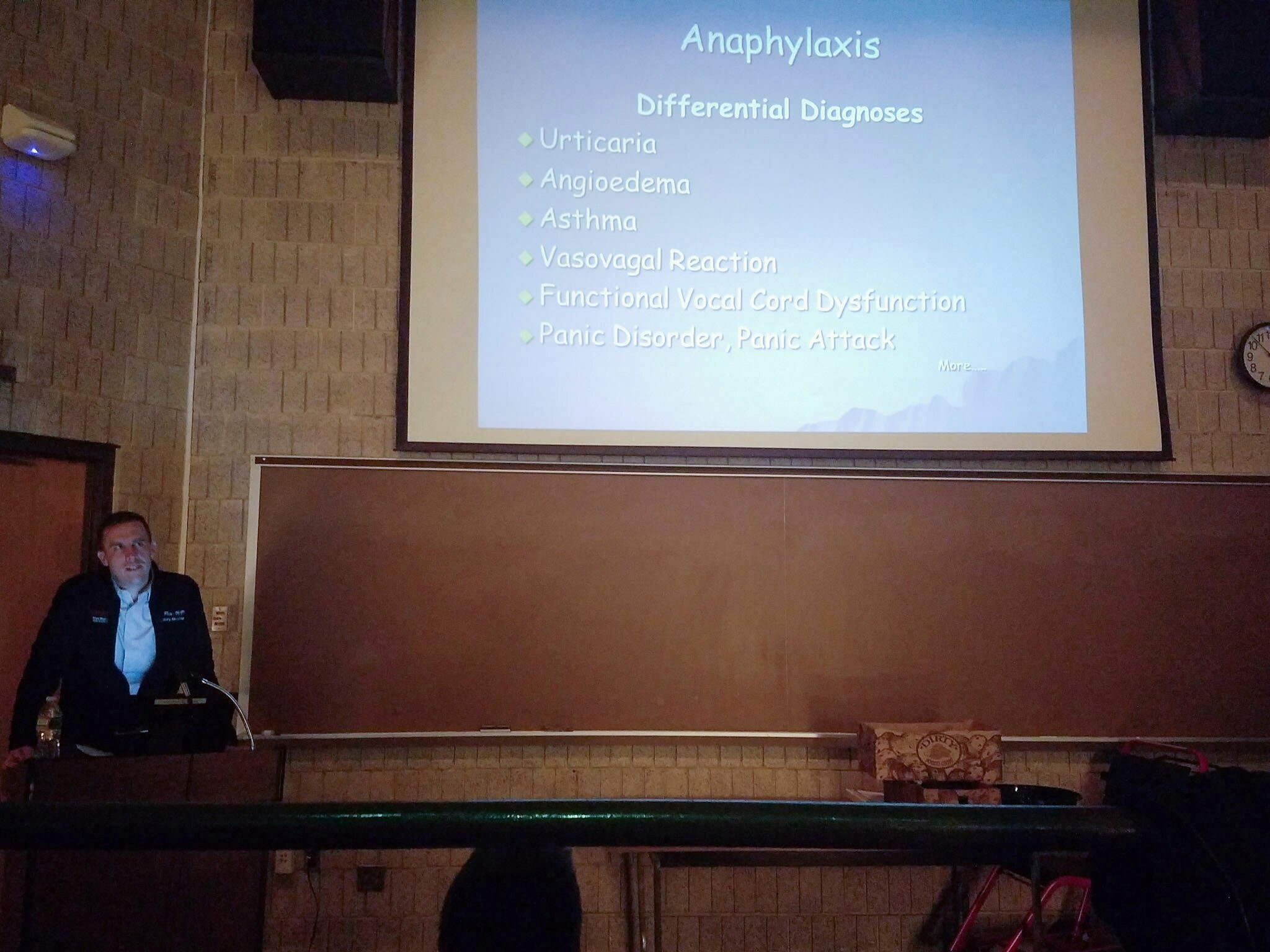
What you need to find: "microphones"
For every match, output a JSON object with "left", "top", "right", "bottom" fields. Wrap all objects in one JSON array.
[{"left": 177, "top": 664, "right": 255, "bottom": 751}]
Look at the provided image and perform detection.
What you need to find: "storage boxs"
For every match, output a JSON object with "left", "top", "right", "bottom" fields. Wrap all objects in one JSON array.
[{"left": 856, "top": 720, "right": 1003, "bottom": 785}]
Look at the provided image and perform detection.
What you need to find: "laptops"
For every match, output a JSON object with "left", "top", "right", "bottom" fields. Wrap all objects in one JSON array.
[{"left": 115, "top": 692, "right": 234, "bottom": 755}]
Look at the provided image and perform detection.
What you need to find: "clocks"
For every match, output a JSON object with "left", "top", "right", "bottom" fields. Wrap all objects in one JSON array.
[{"left": 1238, "top": 323, "right": 1270, "bottom": 390}]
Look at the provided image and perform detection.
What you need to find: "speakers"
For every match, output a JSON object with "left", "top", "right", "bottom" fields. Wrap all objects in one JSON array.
[
  {"left": 251, "top": 0, "right": 399, "bottom": 101},
  {"left": 1149, "top": 0, "right": 1270, "bottom": 140}
]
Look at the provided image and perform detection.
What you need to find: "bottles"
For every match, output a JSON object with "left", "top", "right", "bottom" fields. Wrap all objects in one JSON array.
[{"left": 37, "top": 696, "right": 61, "bottom": 759}]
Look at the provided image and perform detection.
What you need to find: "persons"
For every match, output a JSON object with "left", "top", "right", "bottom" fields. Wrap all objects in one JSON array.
[
  {"left": 1111, "top": 764, "right": 1269, "bottom": 952},
  {"left": 2, "top": 510, "right": 240, "bottom": 769},
  {"left": 438, "top": 827, "right": 582, "bottom": 952}
]
